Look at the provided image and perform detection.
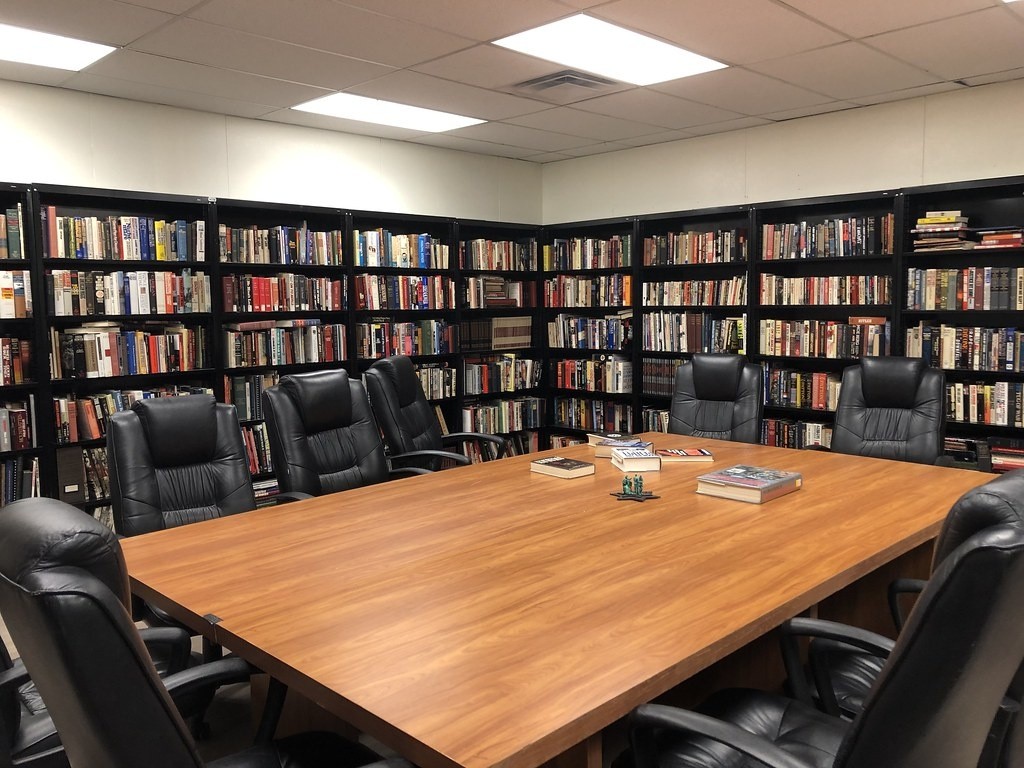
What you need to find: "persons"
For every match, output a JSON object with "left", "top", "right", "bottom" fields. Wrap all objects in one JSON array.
[{"left": 621, "top": 474, "right": 644, "bottom": 497}]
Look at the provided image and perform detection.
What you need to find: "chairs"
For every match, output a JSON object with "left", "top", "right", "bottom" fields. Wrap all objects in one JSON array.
[
  {"left": 262, "top": 369, "right": 436, "bottom": 508},
  {"left": 105, "top": 395, "right": 259, "bottom": 744},
  {"left": 803, "top": 356, "right": 954, "bottom": 471},
  {"left": 670, "top": 353, "right": 763, "bottom": 446},
  {"left": 1, "top": 494, "right": 413, "bottom": 768},
  {"left": 363, "top": 355, "right": 514, "bottom": 470},
  {"left": 779, "top": 466, "right": 1024, "bottom": 767},
  {"left": 1, "top": 622, "right": 289, "bottom": 768},
  {"left": 613, "top": 529, "right": 1023, "bottom": 768}
]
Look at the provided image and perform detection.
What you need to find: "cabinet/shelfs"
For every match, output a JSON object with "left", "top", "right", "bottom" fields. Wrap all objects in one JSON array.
[{"left": 1, "top": 174, "right": 1024, "bottom": 531}]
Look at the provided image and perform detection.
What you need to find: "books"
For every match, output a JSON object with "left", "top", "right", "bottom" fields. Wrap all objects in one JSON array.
[
  {"left": 0, "top": 203, "right": 40, "bottom": 508},
  {"left": 39, "top": 206, "right": 210, "bottom": 534},
  {"left": 587, "top": 431, "right": 661, "bottom": 473},
  {"left": 529, "top": 456, "right": 595, "bottom": 478},
  {"left": 655, "top": 448, "right": 715, "bottom": 462},
  {"left": 903, "top": 211, "right": 1024, "bottom": 428},
  {"left": 353, "top": 228, "right": 456, "bottom": 468},
  {"left": 697, "top": 464, "right": 803, "bottom": 505},
  {"left": 219, "top": 221, "right": 349, "bottom": 508},
  {"left": 759, "top": 213, "right": 894, "bottom": 449},
  {"left": 459, "top": 238, "right": 547, "bottom": 464},
  {"left": 943, "top": 436, "right": 1024, "bottom": 470},
  {"left": 643, "top": 228, "right": 748, "bottom": 434},
  {"left": 543, "top": 235, "right": 631, "bottom": 449}
]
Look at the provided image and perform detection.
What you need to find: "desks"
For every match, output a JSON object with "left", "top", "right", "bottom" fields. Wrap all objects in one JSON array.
[{"left": 117, "top": 431, "right": 1002, "bottom": 768}]
